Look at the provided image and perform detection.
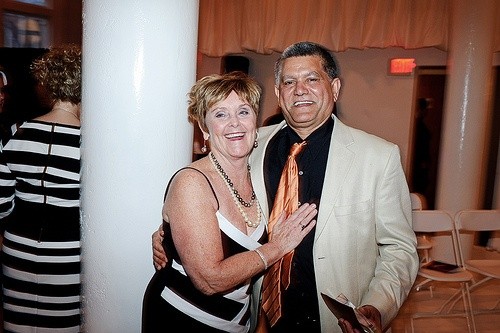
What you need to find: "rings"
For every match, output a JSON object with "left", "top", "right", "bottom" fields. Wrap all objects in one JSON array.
[{"left": 299, "top": 222, "right": 306, "bottom": 229}]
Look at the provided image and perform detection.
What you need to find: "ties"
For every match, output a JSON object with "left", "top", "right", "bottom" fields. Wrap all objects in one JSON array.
[{"left": 260, "top": 140, "right": 307, "bottom": 328}]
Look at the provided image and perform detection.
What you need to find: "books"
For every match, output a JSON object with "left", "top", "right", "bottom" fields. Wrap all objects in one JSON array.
[{"left": 421, "top": 260, "right": 465, "bottom": 273}]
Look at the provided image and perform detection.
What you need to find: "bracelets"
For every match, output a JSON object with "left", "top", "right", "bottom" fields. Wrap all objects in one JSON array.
[{"left": 254, "top": 249, "right": 268, "bottom": 270}]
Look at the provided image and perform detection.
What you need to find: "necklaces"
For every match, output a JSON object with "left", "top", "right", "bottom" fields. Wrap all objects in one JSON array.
[
  {"left": 208, "top": 151, "right": 261, "bottom": 228},
  {"left": 211, "top": 154, "right": 255, "bottom": 207},
  {"left": 50, "top": 106, "right": 79, "bottom": 121}
]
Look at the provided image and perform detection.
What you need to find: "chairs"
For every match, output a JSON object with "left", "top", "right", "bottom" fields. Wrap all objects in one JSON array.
[
  {"left": 454, "top": 211, "right": 500, "bottom": 333},
  {"left": 411, "top": 211, "right": 474, "bottom": 333}
]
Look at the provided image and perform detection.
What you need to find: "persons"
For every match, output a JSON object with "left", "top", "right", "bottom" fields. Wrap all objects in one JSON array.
[
  {"left": 0, "top": 42, "right": 84, "bottom": 333},
  {"left": 142, "top": 71, "right": 318, "bottom": 333},
  {"left": 151, "top": 42, "right": 419, "bottom": 333}
]
